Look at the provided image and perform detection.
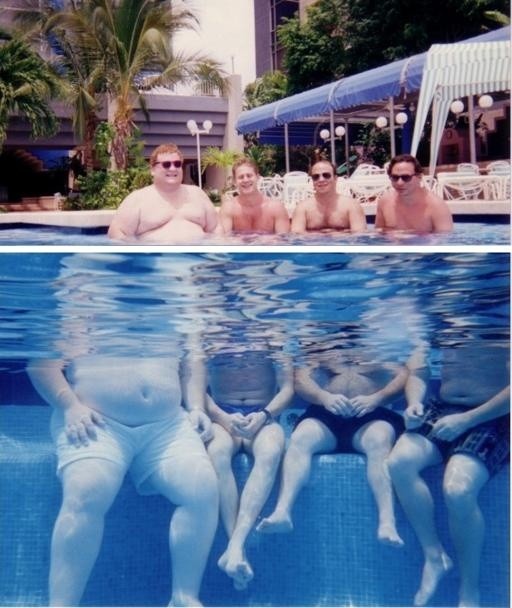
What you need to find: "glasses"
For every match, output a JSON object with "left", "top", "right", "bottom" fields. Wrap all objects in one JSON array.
[
  {"left": 312, "top": 173, "right": 331, "bottom": 181},
  {"left": 389, "top": 173, "right": 416, "bottom": 182},
  {"left": 155, "top": 161, "right": 181, "bottom": 168}
]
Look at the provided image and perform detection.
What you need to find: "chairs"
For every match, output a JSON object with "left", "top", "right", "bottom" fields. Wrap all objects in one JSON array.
[{"left": 221, "top": 159, "right": 511, "bottom": 215}]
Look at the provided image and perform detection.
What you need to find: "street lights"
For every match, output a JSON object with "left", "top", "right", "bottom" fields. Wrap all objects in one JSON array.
[
  {"left": 320, "top": 109, "right": 346, "bottom": 169},
  {"left": 451, "top": 93, "right": 493, "bottom": 164},
  {"left": 375, "top": 96, "right": 408, "bottom": 159},
  {"left": 187, "top": 118, "right": 213, "bottom": 190}
]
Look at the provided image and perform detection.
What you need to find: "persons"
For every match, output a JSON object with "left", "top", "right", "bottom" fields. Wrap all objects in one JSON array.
[
  {"left": 374, "top": 154, "right": 454, "bottom": 242},
  {"left": 27, "top": 354, "right": 222, "bottom": 605},
  {"left": 219, "top": 156, "right": 291, "bottom": 244},
  {"left": 386, "top": 356, "right": 509, "bottom": 605},
  {"left": 256, "top": 354, "right": 407, "bottom": 552},
  {"left": 107, "top": 143, "right": 225, "bottom": 245},
  {"left": 290, "top": 160, "right": 367, "bottom": 243},
  {"left": 205, "top": 354, "right": 294, "bottom": 593}
]
